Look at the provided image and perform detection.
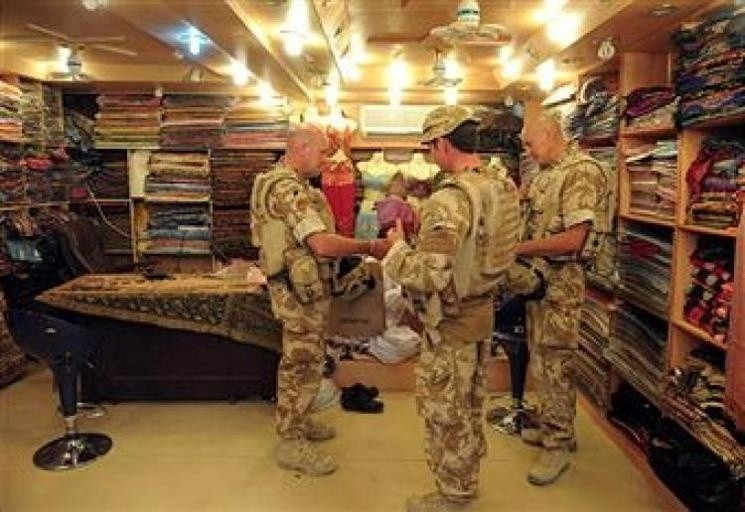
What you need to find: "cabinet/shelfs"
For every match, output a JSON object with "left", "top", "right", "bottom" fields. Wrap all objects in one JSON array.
[
  {"left": 0, "top": 69, "right": 292, "bottom": 390},
  {"left": 579, "top": 0, "right": 744, "bottom": 511}
]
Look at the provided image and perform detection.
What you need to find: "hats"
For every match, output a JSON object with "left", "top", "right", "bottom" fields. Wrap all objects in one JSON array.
[
  {"left": 419, "top": 104, "right": 482, "bottom": 145},
  {"left": 336, "top": 254, "right": 374, "bottom": 301},
  {"left": 506, "top": 254, "right": 553, "bottom": 297}
]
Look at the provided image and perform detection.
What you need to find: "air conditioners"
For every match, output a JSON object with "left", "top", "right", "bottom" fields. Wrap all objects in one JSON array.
[{"left": 361, "top": 103, "right": 440, "bottom": 137}]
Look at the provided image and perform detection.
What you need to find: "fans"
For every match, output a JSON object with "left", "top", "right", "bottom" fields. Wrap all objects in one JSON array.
[
  {"left": 429, "top": 1, "right": 512, "bottom": 47},
  {"left": 4, "top": 20, "right": 140, "bottom": 89}
]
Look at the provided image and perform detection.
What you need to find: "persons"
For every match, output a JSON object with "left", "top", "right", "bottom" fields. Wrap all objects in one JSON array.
[
  {"left": 378, "top": 103, "right": 523, "bottom": 511},
  {"left": 368, "top": 168, "right": 431, "bottom": 243},
  {"left": 511, "top": 112, "right": 613, "bottom": 483},
  {"left": 246, "top": 121, "right": 395, "bottom": 476}
]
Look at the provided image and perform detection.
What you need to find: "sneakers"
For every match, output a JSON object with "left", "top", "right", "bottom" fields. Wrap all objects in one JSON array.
[
  {"left": 276, "top": 417, "right": 340, "bottom": 475},
  {"left": 520, "top": 424, "right": 581, "bottom": 486},
  {"left": 338, "top": 383, "right": 386, "bottom": 413},
  {"left": 401, "top": 489, "right": 476, "bottom": 512}
]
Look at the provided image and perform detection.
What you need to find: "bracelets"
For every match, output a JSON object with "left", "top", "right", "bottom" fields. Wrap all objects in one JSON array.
[{"left": 368, "top": 239, "right": 378, "bottom": 257}]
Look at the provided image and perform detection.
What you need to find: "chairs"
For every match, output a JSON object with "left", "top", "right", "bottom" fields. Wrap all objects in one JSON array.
[{"left": 0, "top": 307, "right": 114, "bottom": 473}]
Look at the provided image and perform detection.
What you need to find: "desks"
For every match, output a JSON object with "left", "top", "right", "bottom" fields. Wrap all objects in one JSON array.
[{"left": 35, "top": 271, "right": 280, "bottom": 404}]
